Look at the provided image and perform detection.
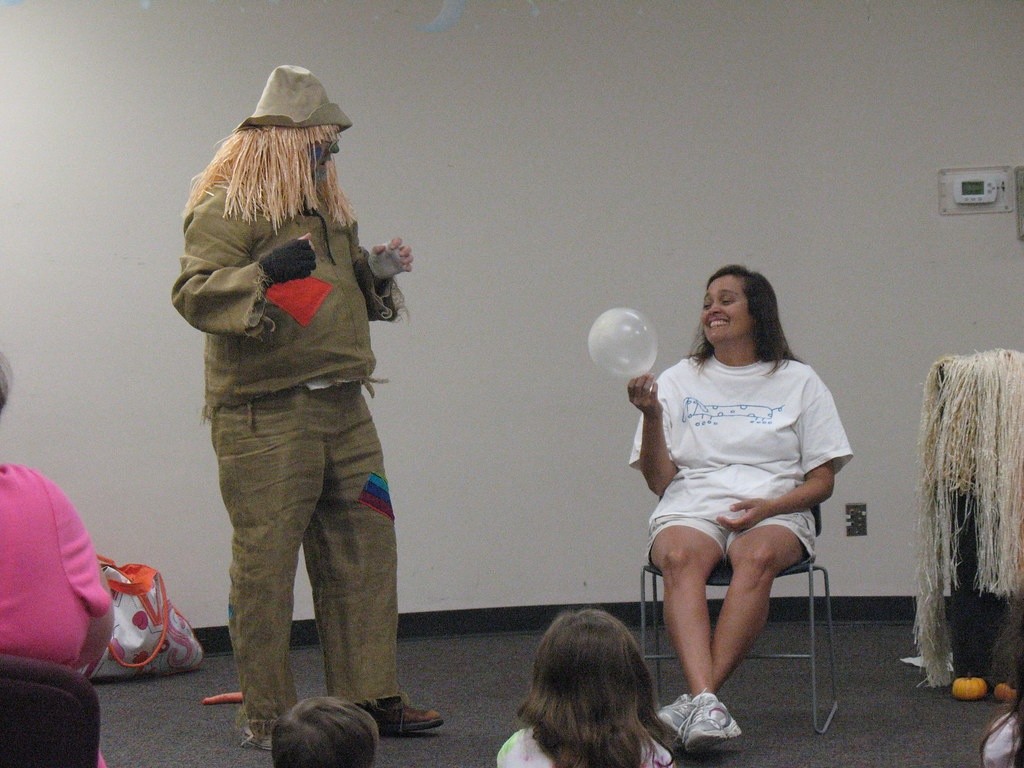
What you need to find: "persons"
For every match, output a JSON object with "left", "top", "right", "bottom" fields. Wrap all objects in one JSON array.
[
  {"left": 168, "top": 66, "right": 445, "bottom": 751},
  {"left": 626, "top": 264, "right": 853, "bottom": 749},
  {"left": 271, "top": 696, "right": 379, "bottom": 768},
  {"left": 979, "top": 583, "right": 1024, "bottom": 768},
  {"left": 495, "top": 607, "right": 678, "bottom": 768},
  {"left": 0, "top": 356, "right": 117, "bottom": 768}
]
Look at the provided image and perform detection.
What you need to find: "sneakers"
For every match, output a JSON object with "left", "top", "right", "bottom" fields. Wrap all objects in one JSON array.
[
  {"left": 653, "top": 693, "right": 694, "bottom": 733},
  {"left": 243, "top": 722, "right": 274, "bottom": 750},
  {"left": 676, "top": 696, "right": 742, "bottom": 752},
  {"left": 357, "top": 698, "right": 445, "bottom": 732}
]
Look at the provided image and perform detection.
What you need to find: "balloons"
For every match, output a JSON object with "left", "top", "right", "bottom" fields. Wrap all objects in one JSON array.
[{"left": 586, "top": 307, "right": 660, "bottom": 378}]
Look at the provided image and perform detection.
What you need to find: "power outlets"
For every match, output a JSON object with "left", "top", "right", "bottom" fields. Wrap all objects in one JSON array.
[{"left": 845, "top": 503, "right": 867, "bottom": 537}]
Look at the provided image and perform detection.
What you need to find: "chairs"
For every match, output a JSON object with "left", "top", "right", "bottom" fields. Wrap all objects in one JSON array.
[{"left": 641, "top": 502, "right": 839, "bottom": 734}]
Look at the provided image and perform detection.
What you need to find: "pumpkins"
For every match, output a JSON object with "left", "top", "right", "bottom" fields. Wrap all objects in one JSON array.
[
  {"left": 951, "top": 672, "right": 988, "bottom": 701},
  {"left": 994, "top": 679, "right": 1017, "bottom": 702}
]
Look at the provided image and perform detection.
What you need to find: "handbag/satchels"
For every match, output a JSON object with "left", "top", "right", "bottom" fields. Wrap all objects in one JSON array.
[{"left": 77, "top": 555, "right": 204, "bottom": 680}]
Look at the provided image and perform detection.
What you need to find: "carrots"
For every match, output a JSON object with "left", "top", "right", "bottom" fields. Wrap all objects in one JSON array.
[{"left": 202, "top": 692, "right": 242, "bottom": 705}]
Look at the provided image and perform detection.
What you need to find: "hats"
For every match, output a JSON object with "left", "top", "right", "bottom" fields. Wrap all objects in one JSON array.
[{"left": 236, "top": 63, "right": 352, "bottom": 132}]
